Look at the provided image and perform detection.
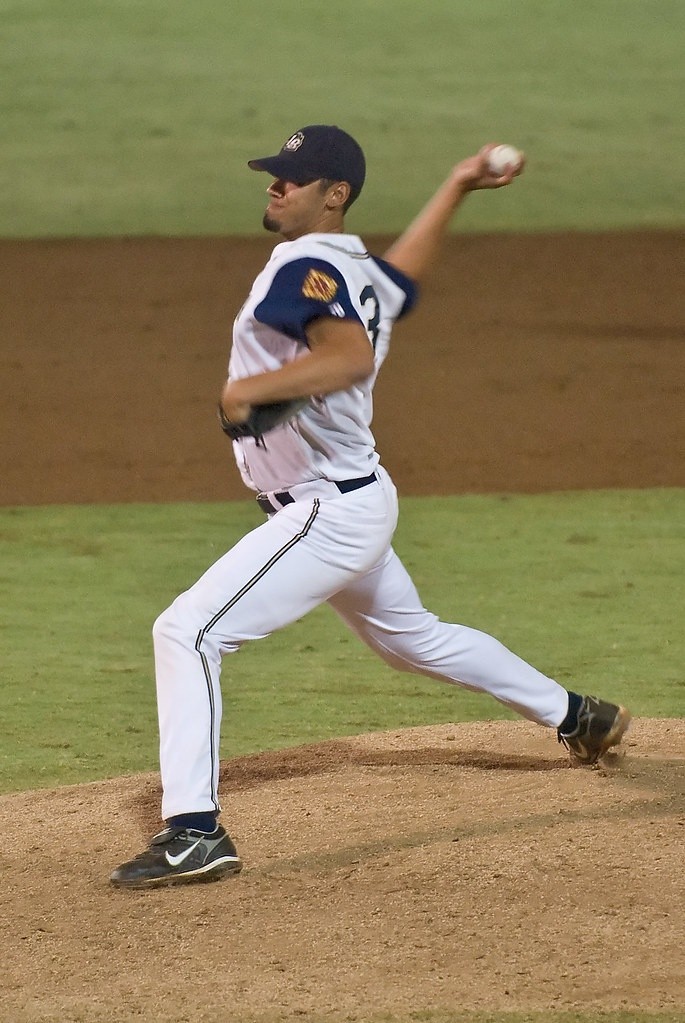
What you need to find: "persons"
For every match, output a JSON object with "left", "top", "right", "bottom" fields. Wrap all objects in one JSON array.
[{"left": 106, "top": 124, "right": 632, "bottom": 889}]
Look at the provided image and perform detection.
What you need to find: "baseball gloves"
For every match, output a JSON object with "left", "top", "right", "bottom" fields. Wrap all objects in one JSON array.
[{"left": 219, "top": 394, "right": 309, "bottom": 439}]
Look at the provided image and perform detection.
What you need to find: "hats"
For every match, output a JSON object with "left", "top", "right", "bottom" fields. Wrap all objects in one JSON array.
[{"left": 247, "top": 124, "right": 367, "bottom": 192}]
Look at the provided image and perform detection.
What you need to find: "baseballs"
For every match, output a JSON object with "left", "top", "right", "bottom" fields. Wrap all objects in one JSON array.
[{"left": 488, "top": 144, "right": 521, "bottom": 174}]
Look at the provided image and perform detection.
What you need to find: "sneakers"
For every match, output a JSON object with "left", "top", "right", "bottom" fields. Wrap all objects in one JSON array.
[
  {"left": 108, "top": 821, "right": 243, "bottom": 889},
  {"left": 558, "top": 695, "right": 631, "bottom": 766}
]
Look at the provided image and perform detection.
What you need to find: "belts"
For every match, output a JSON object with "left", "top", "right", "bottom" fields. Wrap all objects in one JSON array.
[{"left": 255, "top": 473, "right": 377, "bottom": 514}]
